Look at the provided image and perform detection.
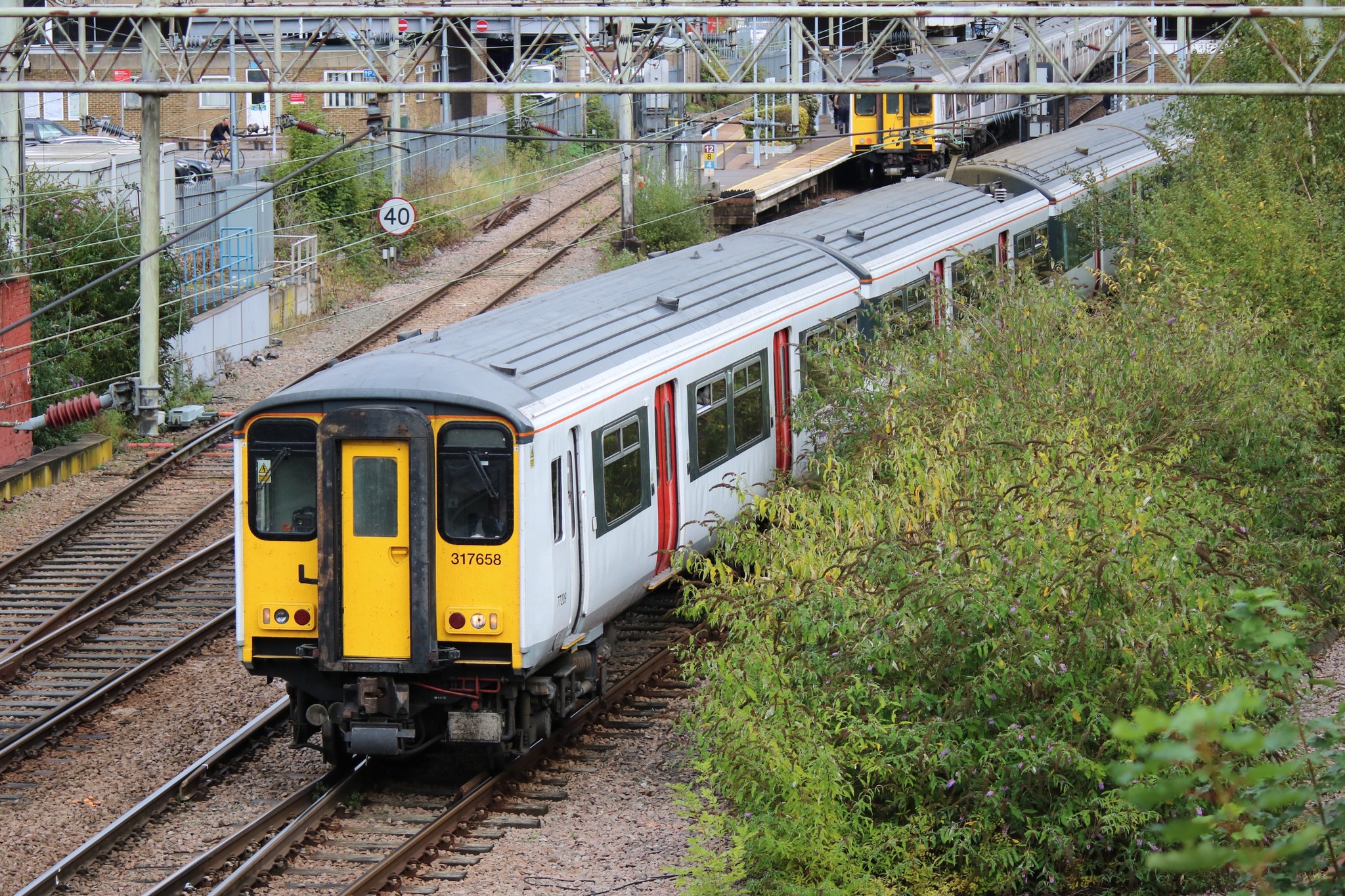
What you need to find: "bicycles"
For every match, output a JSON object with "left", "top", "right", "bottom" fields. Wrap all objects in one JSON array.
[{"left": 204, "top": 138, "right": 245, "bottom": 171}]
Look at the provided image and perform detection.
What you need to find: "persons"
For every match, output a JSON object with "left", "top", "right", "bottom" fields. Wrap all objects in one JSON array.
[
  {"left": 829, "top": 78, "right": 842, "bottom": 129},
  {"left": 210, "top": 118, "right": 240, "bottom": 162},
  {"left": 1102, "top": 94, "right": 1119, "bottom": 117},
  {"left": 834, "top": 94, "right": 850, "bottom": 134}
]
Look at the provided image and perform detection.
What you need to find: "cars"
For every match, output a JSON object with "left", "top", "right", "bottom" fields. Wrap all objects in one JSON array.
[{"left": 174, "top": 153, "right": 213, "bottom": 188}]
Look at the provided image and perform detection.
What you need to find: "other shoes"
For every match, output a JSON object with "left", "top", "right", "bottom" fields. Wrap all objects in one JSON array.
[
  {"left": 224, "top": 158, "right": 231, "bottom": 161},
  {"left": 835, "top": 124, "right": 838, "bottom": 129},
  {"left": 212, "top": 154, "right": 220, "bottom": 160},
  {"left": 840, "top": 126, "right": 843, "bottom": 134}
]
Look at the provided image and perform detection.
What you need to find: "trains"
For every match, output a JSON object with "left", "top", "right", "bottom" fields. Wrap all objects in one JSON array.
[
  {"left": 849, "top": 14, "right": 1133, "bottom": 190},
  {"left": 233, "top": 96, "right": 1204, "bottom": 785}
]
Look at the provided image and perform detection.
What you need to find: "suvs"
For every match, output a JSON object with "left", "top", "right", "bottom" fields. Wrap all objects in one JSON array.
[{"left": 23, "top": 116, "right": 80, "bottom": 157}]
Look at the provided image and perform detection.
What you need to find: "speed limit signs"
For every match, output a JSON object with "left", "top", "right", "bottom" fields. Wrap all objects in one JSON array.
[{"left": 379, "top": 197, "right": 416, "bottom": 236}]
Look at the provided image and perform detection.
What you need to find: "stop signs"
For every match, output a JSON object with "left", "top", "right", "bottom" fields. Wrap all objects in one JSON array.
[
  {"left": 475, "top": 19, "right": 488, "bottom": 33},
  {"left": 582, "top": 47, "right": 594, "bottom": 60},
  {"left": 398, "top": 19, "right": 408, "bottom": 33}
]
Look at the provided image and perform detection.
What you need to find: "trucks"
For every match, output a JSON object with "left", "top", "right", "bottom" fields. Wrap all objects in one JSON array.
[{"left": 507, "top": 57, "right": 568, "bottom": 103}]
